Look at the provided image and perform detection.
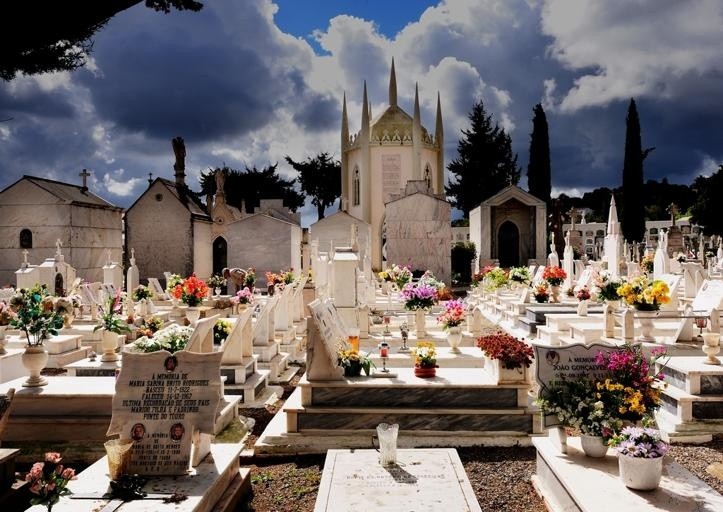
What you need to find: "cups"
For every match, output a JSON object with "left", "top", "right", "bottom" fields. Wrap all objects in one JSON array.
[{"left": 371, "top": 422, "right": 401, "bottom": 467}]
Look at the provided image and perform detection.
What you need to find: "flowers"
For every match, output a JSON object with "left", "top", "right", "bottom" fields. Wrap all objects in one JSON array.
[
  {"left": 336, "top": 261, "right": 535, "bottom": 377},
  {"left": 0, "top": 282, "right": 72, "bottom": 345},
  {"left": 532, "top": 340, "right": 673, "bottom": 457},
  {"left": 537, "top": 249, "right": 714, "bottom": 304},
  {"left": 25, "top": 452, "right": 78, "bottom": 512},
  {"left": 93, "top": 263, "right": 313, "bottom": 353}
]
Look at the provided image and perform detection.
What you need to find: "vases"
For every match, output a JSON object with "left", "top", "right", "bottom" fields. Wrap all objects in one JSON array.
[
  {"left": 99, "top": 330, "right": 119, "bottom": 362},
  {"left": 22, "top": 346, "right": 49, "bottom": 387},
  {"left": 619, "top": 457, "right": 662, "bottom": 492}
]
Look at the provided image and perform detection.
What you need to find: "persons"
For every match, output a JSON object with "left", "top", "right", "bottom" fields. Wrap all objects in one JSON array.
[
  {"left": 170, "top": 422, "right": 185, "bottom": 441},
  {"left": 165, "top": 357, "right": 178, "bottom": 371},
  {"left": 131, "top": 424, "right": 145, "bottom": 441}
]
